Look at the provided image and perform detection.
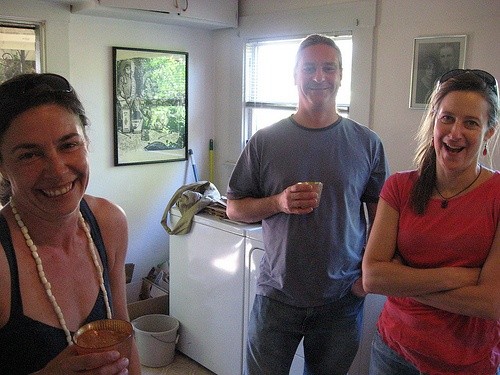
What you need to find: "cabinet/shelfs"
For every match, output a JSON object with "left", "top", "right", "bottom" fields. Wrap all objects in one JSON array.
[{"left": 70, "top": 0, "right": 239, "bottom": 31}]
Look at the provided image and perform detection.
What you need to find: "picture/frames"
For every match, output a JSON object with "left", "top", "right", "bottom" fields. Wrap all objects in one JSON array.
[
  {"left": 408, "top": 34, "right": 468, "bottom": 111},
  {"left": 112, "top": 46, "right": 189, "bottom": 167}
]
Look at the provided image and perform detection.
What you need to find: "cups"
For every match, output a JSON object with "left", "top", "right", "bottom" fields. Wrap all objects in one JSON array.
[
  {"left": 297, "top": 181, "right": 323, "bottom": 208},
  {"left": 72, "top": 319, "right": 141, "bottom": 375}
]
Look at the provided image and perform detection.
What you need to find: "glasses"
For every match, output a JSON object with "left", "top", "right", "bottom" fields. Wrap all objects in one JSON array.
[
  {"left": 0, "top": 73, "right": 74, "bottom": 97},
  {"left": 438, "top": 69, "right": 498, "bottom": 96}
]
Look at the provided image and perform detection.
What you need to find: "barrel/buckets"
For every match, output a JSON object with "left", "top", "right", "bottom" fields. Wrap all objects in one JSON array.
[{"left": 131, "top": 313, "right": 180, "bottom": 368}]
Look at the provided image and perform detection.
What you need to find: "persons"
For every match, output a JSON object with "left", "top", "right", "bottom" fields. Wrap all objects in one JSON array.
[
  {"left": 0, "top": 73, "right": 142, "bottom": 375},
  {"left": 225, "top": 32, "right": 387, "bottom": 375},
  {"left": 361, "top": 68, "right": 500, "bottom": 375}
]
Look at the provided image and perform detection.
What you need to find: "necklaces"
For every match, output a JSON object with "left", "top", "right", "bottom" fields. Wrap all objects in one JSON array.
[
  {"left": 9, "top": 196, "right": 113, "bottom": 347},
  {"left": 435, "top": 165, "right": 482, "bottom": 209}
]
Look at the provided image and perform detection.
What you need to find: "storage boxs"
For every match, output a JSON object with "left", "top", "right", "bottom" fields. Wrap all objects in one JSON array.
[{"left": 126, "top": 273, "right": 171, "bottom": 323}]
